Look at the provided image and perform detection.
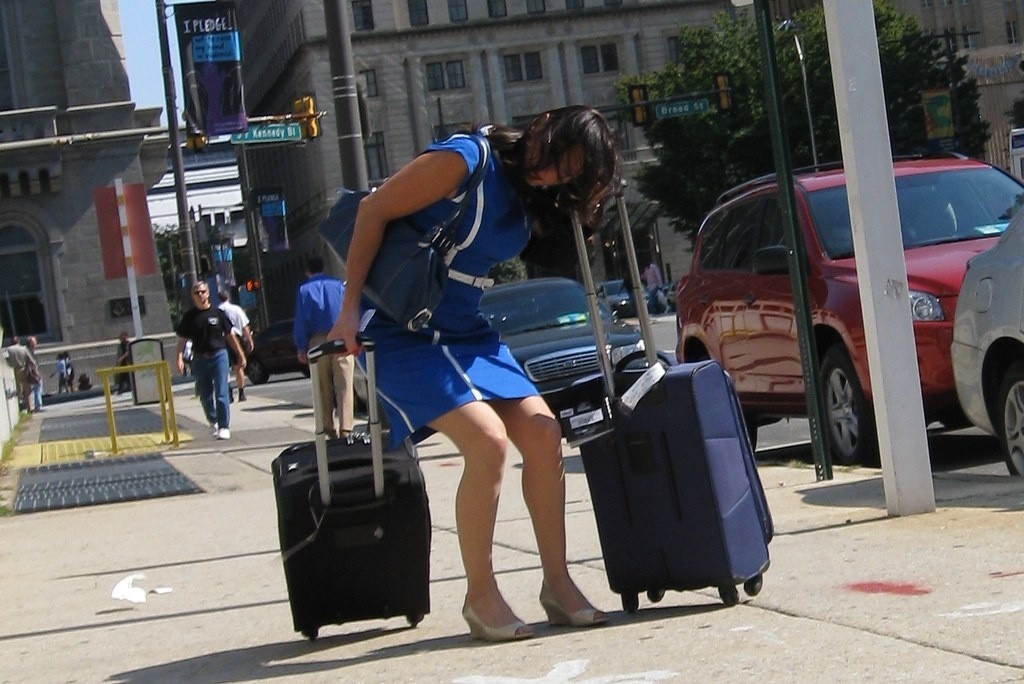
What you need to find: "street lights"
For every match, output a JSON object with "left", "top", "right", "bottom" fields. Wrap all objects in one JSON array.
[{"left": 776, "top": 20, "right": 819, "bottom": 172}]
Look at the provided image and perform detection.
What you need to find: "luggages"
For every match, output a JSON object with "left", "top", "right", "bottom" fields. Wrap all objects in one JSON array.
[
  {"left": 271, "top": 334, "right": 431, "bottom": 641},
  {"left": 569, "top": 178, "right": 773, "bottom": 613}
]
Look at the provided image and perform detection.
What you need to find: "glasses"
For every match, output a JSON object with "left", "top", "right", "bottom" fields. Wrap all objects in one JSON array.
[{"left": 194, "top": 290, "right": 207, "bottom": 294}]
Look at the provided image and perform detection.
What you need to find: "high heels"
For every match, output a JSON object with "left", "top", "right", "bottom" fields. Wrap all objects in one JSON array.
[
  {"left": 540, "top": 580, "right": 610, "bottom": 625},
  {"left": 462, "top": 595, "right": 534, "bottom": 640}
]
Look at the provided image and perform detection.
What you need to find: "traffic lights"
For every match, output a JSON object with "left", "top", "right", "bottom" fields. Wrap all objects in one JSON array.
[{"left": 248, "top": 280, "right": 260, "bottom": 291}]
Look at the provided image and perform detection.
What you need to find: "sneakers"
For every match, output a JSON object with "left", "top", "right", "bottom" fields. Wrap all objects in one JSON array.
[
  {"left": 218, "top": 428, "right": 230, "bottom": 439},
  {"left": 211, "top": 422, "right": 219, "bottom": 437}
]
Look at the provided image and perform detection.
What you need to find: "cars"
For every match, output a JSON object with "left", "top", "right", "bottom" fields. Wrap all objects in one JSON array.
[
  {"left": 243, "top": 318, "right": 313, "bottom": 384},
  {"left": 950, "top": 195, "right": 1024, "bottom": 477},
  {"left": 475, "top": 277, "right": 646, "bottom": 438},
  {"left": 596, "top": 281, "right": 652, "bottom": 317}
]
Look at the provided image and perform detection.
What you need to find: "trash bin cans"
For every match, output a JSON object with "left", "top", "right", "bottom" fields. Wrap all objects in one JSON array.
[{"left": 126, "top": 338, "right": 167, "bottom": 405}]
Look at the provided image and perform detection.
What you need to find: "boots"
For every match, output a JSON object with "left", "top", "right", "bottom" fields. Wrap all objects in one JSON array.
[
  {"left": 239, "top": 387, "right": 246, "bottom": 401},
  {"left": 229, "top": 387, "right": 234, "bottom": 403}
]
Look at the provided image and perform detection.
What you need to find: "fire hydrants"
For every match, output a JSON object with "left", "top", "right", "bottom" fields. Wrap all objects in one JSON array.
[{"left": 77, "top": 373, "right": 93, "bottom": 390}]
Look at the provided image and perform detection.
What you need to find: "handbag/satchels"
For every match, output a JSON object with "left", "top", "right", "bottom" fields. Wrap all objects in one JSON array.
[{"left": 317, "top": 131, "right": 490, "bottom": 334}]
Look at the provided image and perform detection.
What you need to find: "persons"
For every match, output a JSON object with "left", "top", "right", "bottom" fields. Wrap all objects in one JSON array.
[
  {"left": 618, "top": 271, "right": 635, "bottom": 302},
  {"left": 183, "top": 339, "right": 193, "bottom": 364},
  {"left": 6, "top": 335, "right": 74, "bottom": 413},
  {"left": 642, "top": 259, "right": 670, "bottom": 317},
  {"left": 295, "top": 258, "right": 355, "bottom": 439},
  {"left": 115, "top": 332, "right": 132, "bottom": 397},
  {"left": 217, "top": 291, "right": 254, "bottom": 404},
  {"left": 176, "top": 281, "right": 247, "bottom": 439},
  {"left": 329, "top": 104, "right": 618, "bottom": 643}
]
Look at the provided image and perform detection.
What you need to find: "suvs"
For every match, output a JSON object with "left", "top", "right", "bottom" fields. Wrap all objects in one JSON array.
[{"left": 676, "top": 150, "right": 1024, "bottom": 464}]
